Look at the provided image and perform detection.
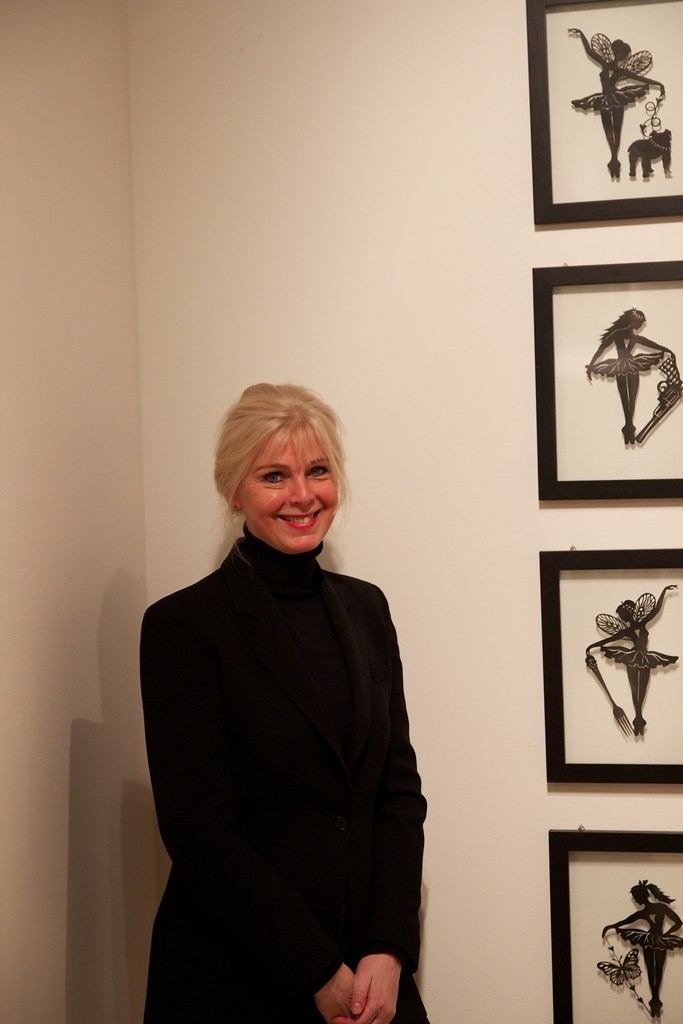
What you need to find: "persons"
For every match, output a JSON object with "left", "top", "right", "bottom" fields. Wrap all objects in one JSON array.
[{"left": 140, "top": 378, "right": 431, "bottom": 1023}]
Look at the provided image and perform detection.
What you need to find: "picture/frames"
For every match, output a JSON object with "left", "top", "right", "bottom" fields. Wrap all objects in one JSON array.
[
  {"left": 526, "top": 0, "right": 683, "bottom": 225},
  {"left": 548, "top": 829, "right": 683, "bottom": 1024},
  {"left": 533, "top": 261, "right": 683, "bottom": 500},
  {"left": 539, "top": 549, "right": 683, "bottom": 784}
]
